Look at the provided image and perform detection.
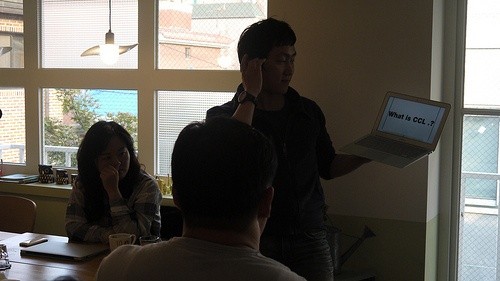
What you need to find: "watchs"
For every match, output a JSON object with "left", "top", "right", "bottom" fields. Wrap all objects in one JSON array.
[{"left": 238, "top": 90, "right": 257, "bottom": 105}]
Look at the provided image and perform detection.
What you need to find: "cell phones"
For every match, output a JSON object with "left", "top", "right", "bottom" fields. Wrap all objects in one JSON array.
[{"left": 19, "top": 237, "right": 48, "bottom": 247}]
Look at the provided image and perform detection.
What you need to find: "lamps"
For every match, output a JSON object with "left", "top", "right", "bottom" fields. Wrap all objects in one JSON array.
[{"left": 99, "top": 0, "right": 120, "bottom": 62}]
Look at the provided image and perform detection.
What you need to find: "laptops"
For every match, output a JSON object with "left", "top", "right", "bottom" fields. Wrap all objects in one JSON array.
[
  {"left": 338, "top": 90, "right": 451, "bottom": 169},
  {"left": 20, "top": 240, "right": 110, "bottom": 262}
]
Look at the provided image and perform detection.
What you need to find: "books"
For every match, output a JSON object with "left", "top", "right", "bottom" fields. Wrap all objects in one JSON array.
[{"left": 0, "top": 173, "right": 39, "bottom": 184}]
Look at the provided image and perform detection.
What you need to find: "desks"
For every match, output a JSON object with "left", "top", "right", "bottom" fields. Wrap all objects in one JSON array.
[{"left": 0, "top": 231, "right": 111, "bottom": 281}]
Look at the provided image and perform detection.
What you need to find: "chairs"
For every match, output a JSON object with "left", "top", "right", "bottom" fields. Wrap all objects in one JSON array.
[
  {"left": 160, "top": 205, "right": 184, "bottom": 240},
  {"left": 0, "top": 195, "right": 37, "bottom": 234}
]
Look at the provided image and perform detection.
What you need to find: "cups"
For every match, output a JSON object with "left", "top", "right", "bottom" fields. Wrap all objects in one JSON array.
[
  {"left": 140, "top": 235, "right": 159, "bottom": 246},
  {"left": 56, "top": 168, "right": 69, "bottom": 185},
  {"left": 109, "top": 233, "right": 136, "bottom": 252},
  {"left": 38, "top": 164, "right": 53, "bottom": 183},
  {"left": 71, "top": 173, "right": 78, "bottom": 186},
  {"left": 0, "top": 244, "right": 11, "bottom": 270}
]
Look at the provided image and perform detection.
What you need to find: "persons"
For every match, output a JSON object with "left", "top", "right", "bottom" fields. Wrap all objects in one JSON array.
[
  {"left": 97, "top": 116, "right": 306, "bottom": 281},
  {"left": 206, "top": 18, "right": 373, "bottom": 281},
  {"left": 65, "top": 121, "right": 163, "bottom": 244}
]
